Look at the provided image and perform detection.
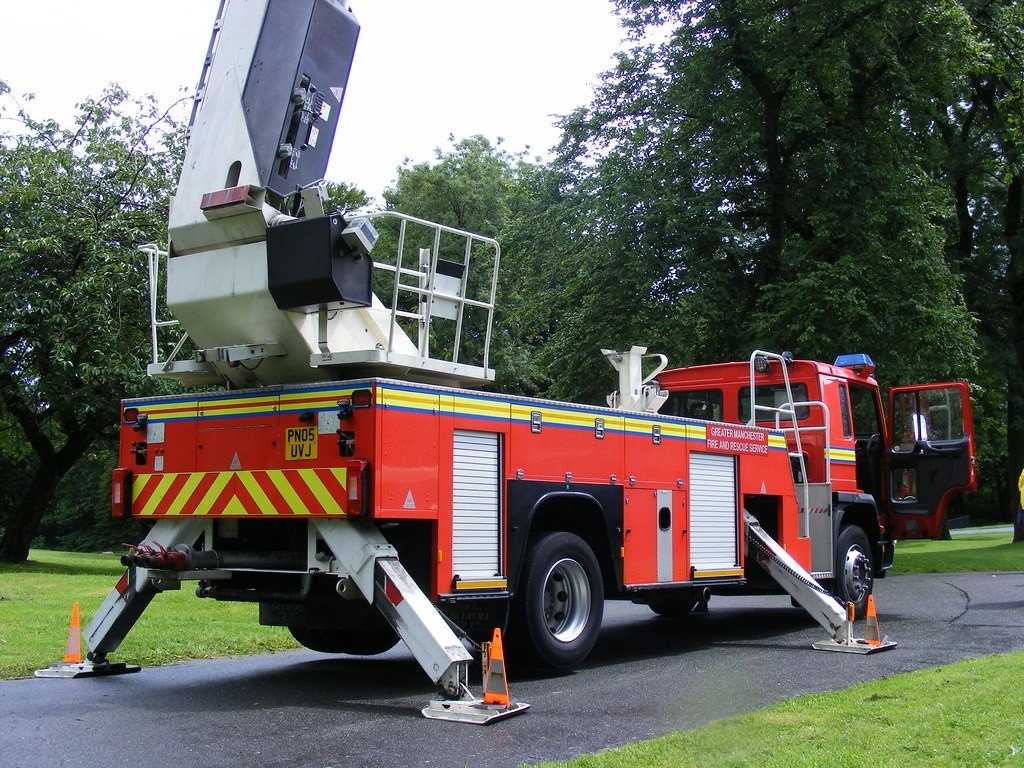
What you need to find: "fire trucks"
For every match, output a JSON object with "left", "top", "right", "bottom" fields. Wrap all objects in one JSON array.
[{"left": 35, "top": 1, "right": 974, "bottom": 726}]
